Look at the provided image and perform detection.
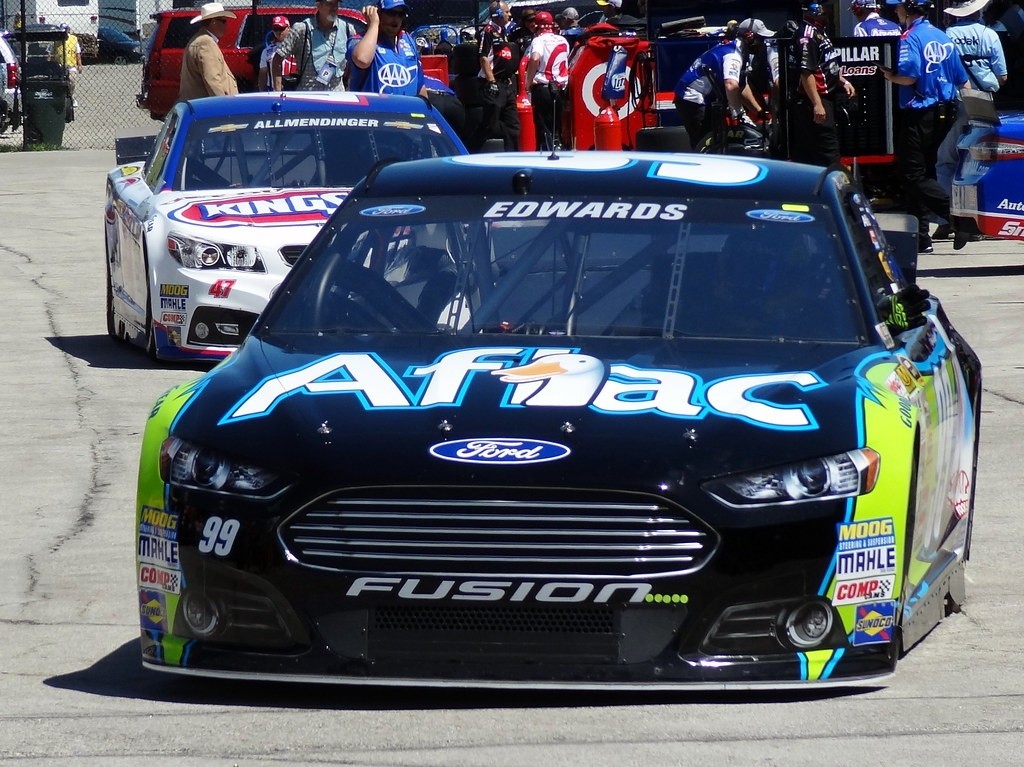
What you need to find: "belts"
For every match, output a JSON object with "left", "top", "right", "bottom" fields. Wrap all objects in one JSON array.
[{"left": 543, "top": 84, "right": 549, "bottom": 87}]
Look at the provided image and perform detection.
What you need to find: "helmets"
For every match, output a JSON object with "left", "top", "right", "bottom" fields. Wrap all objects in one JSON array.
[
  {"left": 802, "top": 0, "right": 835, "bottom": 31},
  {"left": 533, "top": 11, "right": 555, "bottom": 29},
  {"left": 715, "top": 227, "right": 813, "bottom": 323}
]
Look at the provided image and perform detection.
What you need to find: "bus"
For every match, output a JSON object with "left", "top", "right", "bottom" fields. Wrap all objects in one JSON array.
[{"left": 35, "top": 0, "right": 99, "bottom": 58}]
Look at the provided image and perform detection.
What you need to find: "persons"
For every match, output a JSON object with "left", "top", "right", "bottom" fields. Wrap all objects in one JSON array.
[
  {"left": 12, "top": 0, "right": 620, "bottom": 153},
  {"left": 676, "top": 0, "right": 1024, "bottom": 251}
]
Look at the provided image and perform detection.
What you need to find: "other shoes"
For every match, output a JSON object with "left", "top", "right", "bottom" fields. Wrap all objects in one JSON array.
[
  {"left": 918, "top": 240, "right": 933, "bottom": 252},
  {"left": 953, "top": 213, "right": 975, "bottom": 250}
]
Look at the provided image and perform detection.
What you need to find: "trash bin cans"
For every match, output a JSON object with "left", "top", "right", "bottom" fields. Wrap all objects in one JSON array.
[{"left": 14, "top": 22, "right": 75, "bottom": 152}]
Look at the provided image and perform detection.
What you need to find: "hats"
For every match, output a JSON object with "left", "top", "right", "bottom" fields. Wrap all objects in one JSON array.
[
  {"left": 596, "top": 0, "right": 622, "bottom": 7},
  {"left": 190, "top": 3, "right": 237, "bottom": 24},
  {"left": 846, "top": 0, "right": 877, "bottom": 12},
  {"left": 272, "top": 16, "right": 290, "bottom": 27},
  {"left": 555, "top": 7, "right": 579, "bottom": 20},
  {"left": 376, "top": 0, "right": 408, "bottom": 11},
  {"left": 943, "top": 0, "right": 990, "bottom": 17},
  {"left": 17, "top": 11, "right": 21, "bottom": 16},
  {"left": 740, "top": 18, "right": 776, "bottom": 37},
  {"left": 60, "top": 23, "right": 69, "bottom": 30},
  {"left": 885, "top": 0, "right": 930, "bottom": 7}
]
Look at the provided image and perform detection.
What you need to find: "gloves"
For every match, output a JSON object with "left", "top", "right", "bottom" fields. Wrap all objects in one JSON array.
[
  {"left": 486, "top": 80, "right": 502, "bottom": 102},
  {"left": 757, "top": 109, "right": 779, "bottom": 125}
]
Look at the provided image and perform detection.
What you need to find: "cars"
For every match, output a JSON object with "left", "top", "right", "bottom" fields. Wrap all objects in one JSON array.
[
  {"left": 410, "top": 23, "right": 477, "bottom": 48},
  {"left": 565, "top": 11, "right": 608, "bottom": 35},
  {"left": 134, "top": 150, "right": 983, "bottom": 692},
  {"left": 935, "top": 110, "right": 1024, "bottom": 241},
  {"left": 0, "top": 32, "right": 23, "bottom": 134},
  {"left": 97, "top": 26, "right": 146, "bottom": 64},
  {"left": 103, "top": 90, "right": 497, "bottom": 359}
]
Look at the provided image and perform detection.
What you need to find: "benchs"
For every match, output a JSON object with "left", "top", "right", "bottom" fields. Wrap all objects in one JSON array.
[
  {"left": 488, "top": 223, "right": 670, "bottom": 333},
  {"left": 199, "top": 149, "right": 318, "bottom": 188}
]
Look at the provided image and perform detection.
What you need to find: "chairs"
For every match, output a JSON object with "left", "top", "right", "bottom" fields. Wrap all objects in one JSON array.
[
  {"left": 309, "top": 130, "right": 428, "bottom": 188},
  {"left": 649, "top": 227, "right": 859, "bottom": 342}
]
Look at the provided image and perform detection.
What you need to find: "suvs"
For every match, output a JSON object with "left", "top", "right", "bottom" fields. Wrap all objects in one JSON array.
[{"left": 136, "top": 5, "right": 449, "bottom": 123}]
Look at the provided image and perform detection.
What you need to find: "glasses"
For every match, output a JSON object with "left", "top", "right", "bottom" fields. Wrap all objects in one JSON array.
[
  {"left": 383, "top": 6, "right": 407, "bottom": 19},
  {"left": 323, "top": 0, "right": 339, "bottom": 8},
  {"left": 273, "top": 28, "right": 285, "bottom": 32},
  {"left": 215, "top": 18, "right": 226, "bottom": 24}
]
